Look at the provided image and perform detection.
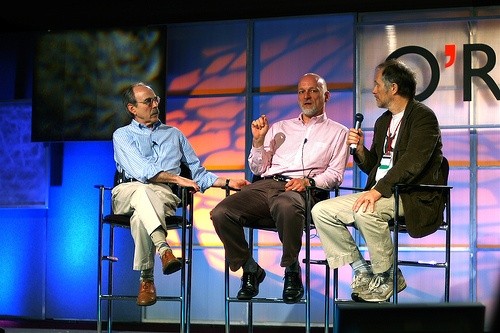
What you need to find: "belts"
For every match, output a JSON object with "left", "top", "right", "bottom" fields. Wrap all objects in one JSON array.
[
  {"left": 261, "top": 173, "right": 294, "bottom": 183},
  {"left": 124, "top": 177, "right": 173, "bottom": 188}
]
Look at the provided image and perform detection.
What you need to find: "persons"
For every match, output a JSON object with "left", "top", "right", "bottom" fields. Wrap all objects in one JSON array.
[
  {"left": 111, "top": 83, "right": 252, "bottom": 306},
  {"left": 313, "top": 60, "right": 444, "bottom": 304},
  {"left": 210, "top": 72, "right": 353, "bottom": 304}
]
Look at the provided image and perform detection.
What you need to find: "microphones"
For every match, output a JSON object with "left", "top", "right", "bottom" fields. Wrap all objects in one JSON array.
[
  {"left": 303, "top": 138, "right": 308, "bottom": 145},
  {"left": 350, "top": 113, "right": 364, "bottom": 154}
]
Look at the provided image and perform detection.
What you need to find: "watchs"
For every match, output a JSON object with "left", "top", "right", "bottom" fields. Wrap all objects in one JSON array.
[{"left": 307, "top": 177, "right": 315, "bottom": 188}]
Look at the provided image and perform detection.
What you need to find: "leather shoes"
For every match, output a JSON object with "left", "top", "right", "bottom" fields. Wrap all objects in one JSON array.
[
  {"left": 237, "top": 263, "right": 266, "bottom": 299},
  {"left": 161, "top": 250, "right": 182, "bottom": 275},
  {"left": 283, "top": 266, "right": 304, "bottom": 305},
  {"left": 136, "top": 279, "right": 156, "bottom": 306}
]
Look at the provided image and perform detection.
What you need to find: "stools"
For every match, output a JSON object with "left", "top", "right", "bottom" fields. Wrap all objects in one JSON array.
[
  {"left": 222, "top": 180, "right": 340, "bottom": 333},
  {"left": 332, "top": 155, "right": 454, "bottom": 333},
  {"left": 93, "top": 163, "right": 195, "bottom": 333}
]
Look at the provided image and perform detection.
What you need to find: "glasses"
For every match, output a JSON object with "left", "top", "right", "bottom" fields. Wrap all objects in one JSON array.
[{"left": 137, "top": 95, "right": 160, "bottom": 104}]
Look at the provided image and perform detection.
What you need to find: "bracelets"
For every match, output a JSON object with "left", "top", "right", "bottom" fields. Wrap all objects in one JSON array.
[{"left": 225, "top": 179, "right": 230, "bottom": 187}]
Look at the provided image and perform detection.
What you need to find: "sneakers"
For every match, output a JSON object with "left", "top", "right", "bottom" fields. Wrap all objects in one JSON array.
[{"left": 352, "top": 267, "right": 407, "bottom": 302}]
